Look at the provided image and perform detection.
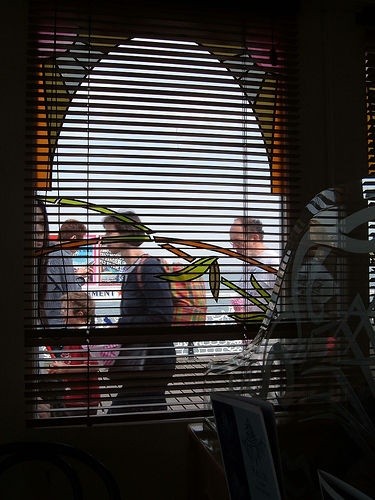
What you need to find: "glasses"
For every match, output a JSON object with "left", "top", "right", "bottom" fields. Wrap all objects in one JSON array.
[{"left": 229, "top": 237, "right": 254, "bottom": 245}]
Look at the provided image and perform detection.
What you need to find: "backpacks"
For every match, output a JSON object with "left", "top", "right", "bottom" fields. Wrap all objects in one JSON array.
[{"left": 138, "top": 255, "right": 206, "bottom": 326}]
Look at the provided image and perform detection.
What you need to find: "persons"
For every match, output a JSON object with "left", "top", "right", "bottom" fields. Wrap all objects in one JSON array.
[
  {"left": 45, "top": 290, "right": 101, "bottom": 417},
  {"left": 44, "top": 218, "right": 97, "bottom": 357},
  {"left": 230, "top": 218, "right": 280, "bottom": 364},
  {"left": 105, "top": 210, "right": 179, "bottom": 416}
]
